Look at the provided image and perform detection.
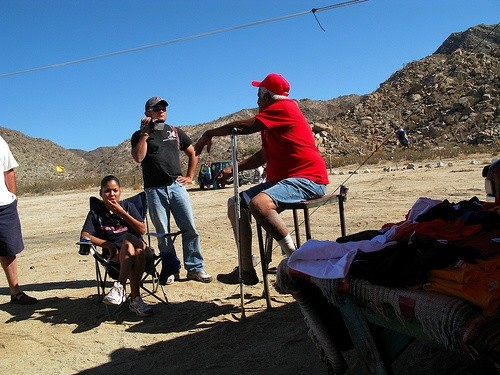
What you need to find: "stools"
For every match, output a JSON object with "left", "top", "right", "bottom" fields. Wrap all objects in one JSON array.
[{"left": 248, "top": 194, "right": 348, "bottom": 308}]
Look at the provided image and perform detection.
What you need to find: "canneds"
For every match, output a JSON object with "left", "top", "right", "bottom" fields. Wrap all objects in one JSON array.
[
  {"left": 79, "top": 237, "right": 91, "bottom": 255},
  {"left": 394, "top": 128, "right": 410, "bottom": 146}
]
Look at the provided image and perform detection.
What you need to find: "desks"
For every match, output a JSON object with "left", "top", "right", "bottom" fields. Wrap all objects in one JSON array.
[{"left": 276, "top": 204, "right": 500, "bottom": 375}]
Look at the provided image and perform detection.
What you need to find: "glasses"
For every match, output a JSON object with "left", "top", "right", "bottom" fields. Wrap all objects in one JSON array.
[{"left": 148, "top": 105, "right": 167, "bottom": 112}]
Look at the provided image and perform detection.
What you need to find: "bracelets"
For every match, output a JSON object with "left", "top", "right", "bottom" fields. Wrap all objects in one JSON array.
[{"left": 140, "top": 132, "right": 149, "bottom": 136}]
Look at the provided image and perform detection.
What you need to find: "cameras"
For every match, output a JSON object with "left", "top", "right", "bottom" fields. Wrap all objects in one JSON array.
[{"left": 149, "top": 119, "right": 165, "bottom": 131}]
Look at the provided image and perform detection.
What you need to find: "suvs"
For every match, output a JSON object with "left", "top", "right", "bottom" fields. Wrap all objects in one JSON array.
[{"left": 198, "top": 159, "right": 244, "bottom": 191}]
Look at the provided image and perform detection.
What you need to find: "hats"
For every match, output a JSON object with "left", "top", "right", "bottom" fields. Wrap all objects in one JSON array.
[
  {"left": 251, "top": 72, "right": 291, "bottom": 96},
  {"left": 145, "top": 96, "right": 168, "bottom": 109}
]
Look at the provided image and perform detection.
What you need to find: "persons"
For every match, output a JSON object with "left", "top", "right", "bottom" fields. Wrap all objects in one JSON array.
[
  {"left": 131, "top": 97, "right": 213, "bottom": 284},
  {"left": 194, "top": 74, "right": 330, "bottom": 294},
  {"left": 0, "top": 134, "right": 38, "bottom": 304},
  {"left": 79, "top": 176, "right": 153, "bottom": 317},
  {"left": 204, "top": 162, "right": 212, "bottom": 189}
]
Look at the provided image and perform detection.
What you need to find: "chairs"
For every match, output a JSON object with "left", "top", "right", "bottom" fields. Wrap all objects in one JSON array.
[{"left": 76, "top": 192, "right": 187, "bottom": 314}]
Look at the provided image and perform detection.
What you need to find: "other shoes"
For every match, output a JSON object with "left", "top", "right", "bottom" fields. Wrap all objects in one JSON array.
[
  {"left": 161, "top": 271, "right": 180, "bottom": 285},
  {"left": 129, "top": 295, "right": 152, "bottom": 317},
  {"left": 10, "top": 292, "right": 37, "bottom": 303},
  {"left": 102, "top": 281, "right": 128, "bottom": 306},
  {"left": 187, "top": 269, "right": 212, "bottom": 283},
  {"left": 217, "top": 267, "right": 259, "bottom": 285}
]
